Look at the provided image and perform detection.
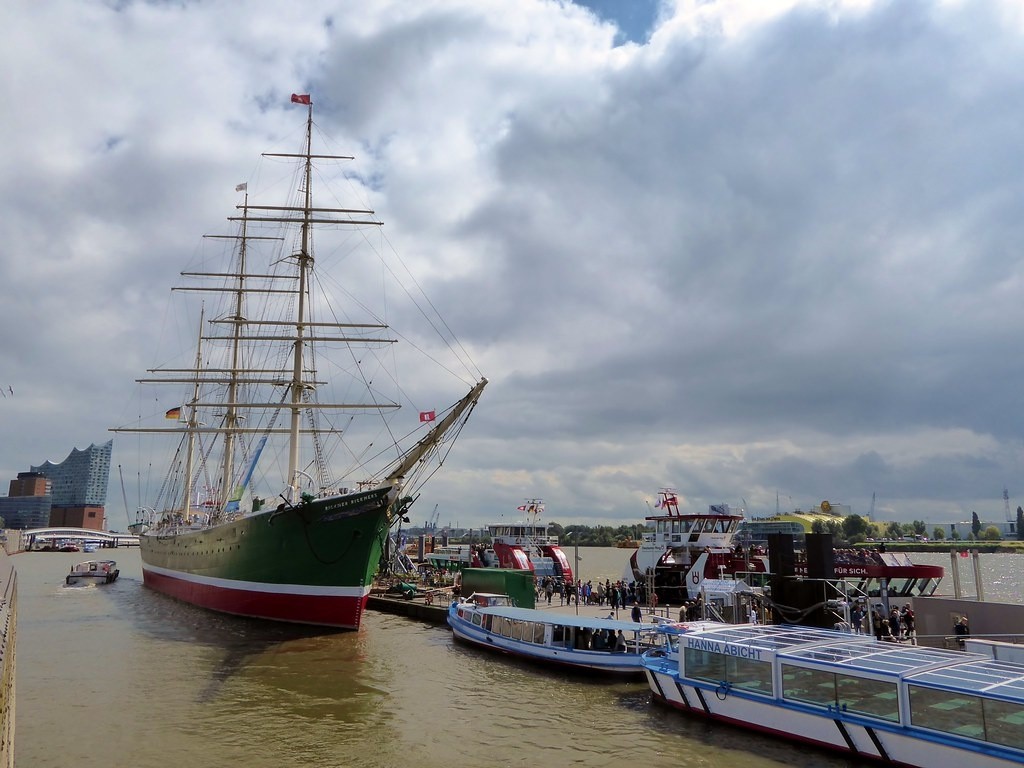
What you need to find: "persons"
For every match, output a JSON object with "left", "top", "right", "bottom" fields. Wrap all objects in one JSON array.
[
  {"left": 679, "top": 597, "right": 702, "bottom": 622},
  {"left": 555, "top": 628, "right": 625, "bottom": 651},
  {"left": 607, "top": 612, "right": 614, "bottom": 620},
  {"left": 734, "top": 543, "right": 742, "bottom": 556},
  {"left": 890, "top": 603, "right": 914, "bottom": 639},
  {"left": 540, "top": 576, "right": 642, "bottom": 610},
  {"left": 873, "top": 611, "right": 889, "bottom": 640},
  {"left": 631, "top": 602, "right": 643, "bottom": 639},
  {"left": 955, "top": 617, "right": 971, "bottom": 651},
  {"left": 750, "top": 544, "right": 768, "bottom": 557},
  {"left": 833, "top": 548, "right": 883, "bottom": 566},
  {"left": 879, "top": 542, "right": 885, "bottom": 553},
  {"left": 852, "top": 606, "right": 863, "bottom": 634}
]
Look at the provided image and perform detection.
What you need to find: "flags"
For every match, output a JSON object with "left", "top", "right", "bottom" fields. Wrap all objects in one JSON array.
[
  {"left": 166, "top": 407, "right": 180, "bottom": 419},
  {"left": 517, "top": 506, "right": 525, "bottom": 511},
  {"left": 420, "top": 411, "right": 435, "bottom": 422},
  {"left": 655, "top": 497, "right": 665, "bottom": 509},
  {"left": 961, "top": 552, "right": 968, "bottom": 557},
  {"left": 528, "top": 506, "right": 534, "bottom": 512}
]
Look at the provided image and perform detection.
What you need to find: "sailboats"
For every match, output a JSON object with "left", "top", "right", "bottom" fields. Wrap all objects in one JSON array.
[{"left": 108, "top": 94, "right": 489, "bottom": 633}]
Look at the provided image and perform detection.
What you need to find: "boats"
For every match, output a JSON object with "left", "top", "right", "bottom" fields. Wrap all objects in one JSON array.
[
  {"left": 620, "top": 486, "right": 948, "bottom": 612},
  {"left": 57, "top": 542, "right": 97, "bottom": 553},
  {"left": 425, "top": 497, "right": 574, "bottom": 586},
  {"left": 445, "top": 588, "right": 669, "bottom": 674},
  {"left": 639, "top": 597, "right": 1024, "bottom": 768},
  {"left": 64, "top": 560, "right": 123, "bottom": 587}
]
